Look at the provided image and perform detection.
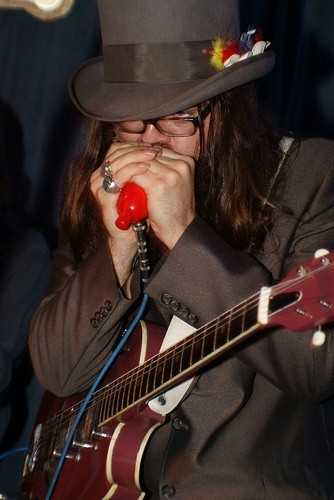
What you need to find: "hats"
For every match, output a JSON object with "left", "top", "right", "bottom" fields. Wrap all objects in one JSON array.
[{"left": 68, "top": 0, "right": 276, "bottom": 122}]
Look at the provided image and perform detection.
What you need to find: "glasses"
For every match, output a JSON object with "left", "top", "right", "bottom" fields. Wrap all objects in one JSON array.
[{"left": 111, "top": 96, "right": 220, "bottom": 138}]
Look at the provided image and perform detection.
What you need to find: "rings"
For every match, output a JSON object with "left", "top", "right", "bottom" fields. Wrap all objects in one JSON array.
[
  {"left": 101, "top": 173, "right": 125, "bottom": 194},
  {"left": 100, "top": 160, "right": 118, "bottom": 177}
]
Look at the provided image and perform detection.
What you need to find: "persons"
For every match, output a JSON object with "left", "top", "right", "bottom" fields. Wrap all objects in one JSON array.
[{"left": 22, "top": 0, "right": 334, "bottom": 500}]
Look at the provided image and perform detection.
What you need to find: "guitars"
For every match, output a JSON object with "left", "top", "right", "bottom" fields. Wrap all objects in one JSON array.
[{"left": 15, "top": 247, "right": 334, "bottom": 500}]
